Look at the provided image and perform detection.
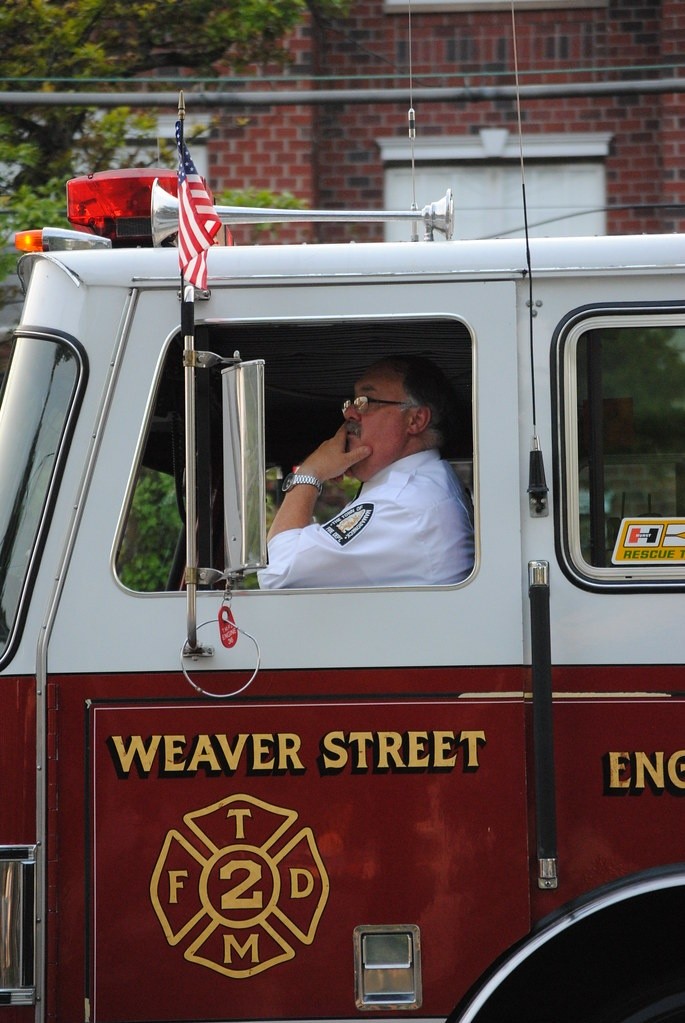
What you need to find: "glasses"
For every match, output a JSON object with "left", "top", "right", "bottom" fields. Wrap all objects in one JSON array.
[{"left": 342, "top": 392, "right": 407, "bottom": 418}]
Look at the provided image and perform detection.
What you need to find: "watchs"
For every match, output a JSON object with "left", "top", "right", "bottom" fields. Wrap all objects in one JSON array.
[{"left": 282, "top": 473, "right": 322, "bottom": 497}]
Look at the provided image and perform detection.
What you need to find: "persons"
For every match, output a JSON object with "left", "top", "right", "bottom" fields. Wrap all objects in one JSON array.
[{"left": 257, "top": 354, "right": 476, "bottom": 589}]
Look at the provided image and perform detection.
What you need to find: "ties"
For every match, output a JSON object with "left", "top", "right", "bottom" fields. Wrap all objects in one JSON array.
[{"left": 351, "top": 481, "right": 364, "bottom": 505}]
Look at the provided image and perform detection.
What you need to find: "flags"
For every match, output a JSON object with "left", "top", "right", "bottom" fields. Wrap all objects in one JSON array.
[{"left": 175, "top": 121, "right": 222, "bottom": 290}]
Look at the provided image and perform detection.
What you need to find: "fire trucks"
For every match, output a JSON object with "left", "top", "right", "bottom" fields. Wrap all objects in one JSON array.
[{"left": 0, "top": 171, "right": 685, "bottom": 1023}]
